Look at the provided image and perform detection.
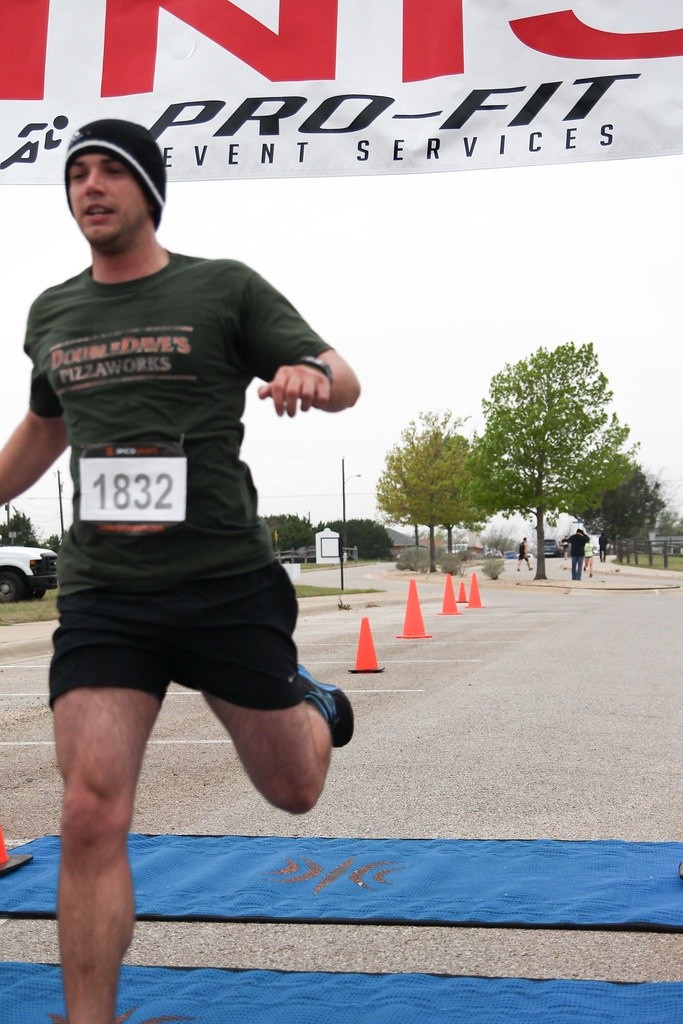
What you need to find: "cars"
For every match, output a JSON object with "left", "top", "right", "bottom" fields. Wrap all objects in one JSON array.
[
  {"left": 542, "top": 539, "right": 571, "bottom": 558},
  {"left": 526, "top": 554, "right": 533, "bottom": 559},
  {"left": 506, "top": 551, "right": 518, "bottom": 560}
]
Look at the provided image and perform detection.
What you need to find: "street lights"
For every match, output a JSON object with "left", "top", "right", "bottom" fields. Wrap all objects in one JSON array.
[{"left": 340, "top": 457, "right": 363, "bottom": 592}]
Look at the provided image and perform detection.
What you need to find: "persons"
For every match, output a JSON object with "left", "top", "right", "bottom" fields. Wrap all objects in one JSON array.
[
  {"left": 518, "top": 537, "right": 533, "bottom": 572},
  {"left": 599, "top": 532, "right": 608, "bottom": 562},
  {"left": 566, "top": 529, "right": 589, "bottom": 580},
  {"left": 562, "top": 535, "right": 569, "bottom": 559},
  {"left": 585, "top": 540, "right": 594, "bottom": 578},
  {"left": 0, "top": 119, "right": 363, "bottom": 1024}
]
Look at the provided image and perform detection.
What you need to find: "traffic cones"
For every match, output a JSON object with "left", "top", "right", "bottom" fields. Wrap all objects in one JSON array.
[
  {"left": 346, "top": 616, "right": 387, "bottom": 673},
  {"left": 394, "top": 578, "right": 434, "bottom": 640},
  {"left": 436, "top": 573, "right": 465, "bottom": 618},
  {"left": 466, "top": 572, "right": 485, "bottom": 608},
  {"left": 455, "top": 581, "right": 469, "bottom": 604}
]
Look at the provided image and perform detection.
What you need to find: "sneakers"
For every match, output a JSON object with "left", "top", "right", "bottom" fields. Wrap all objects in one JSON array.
[{"left": 295, "top": 664, "right": 355, "bottom": 753}]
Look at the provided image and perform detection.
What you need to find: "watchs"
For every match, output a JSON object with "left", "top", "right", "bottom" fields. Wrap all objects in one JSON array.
[{"left": 295, "top": 355, "right": 334, "bottom": 383}]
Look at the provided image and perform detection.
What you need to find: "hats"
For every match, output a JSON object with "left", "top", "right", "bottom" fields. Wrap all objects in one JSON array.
[{"left": 63, "top": 118, "right": 165, "bottom": 230}]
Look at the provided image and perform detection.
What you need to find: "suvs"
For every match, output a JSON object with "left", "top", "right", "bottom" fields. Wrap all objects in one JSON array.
[{"left": 0, "top": 534, "right": 59, "bottom": 603}]
